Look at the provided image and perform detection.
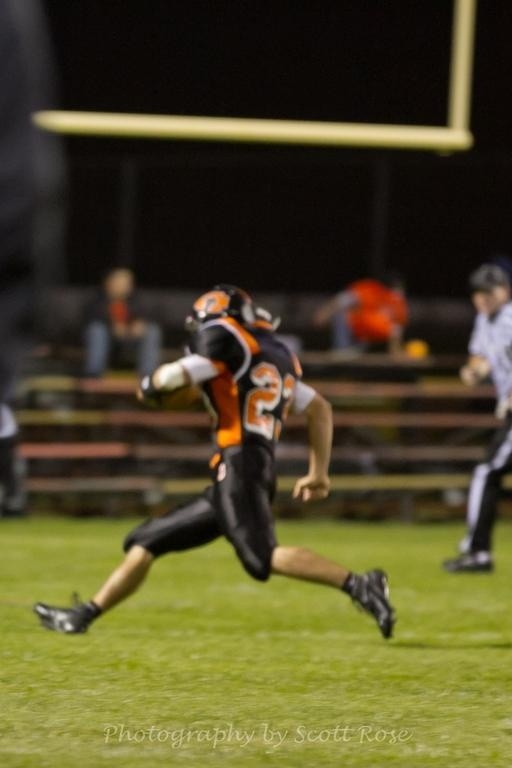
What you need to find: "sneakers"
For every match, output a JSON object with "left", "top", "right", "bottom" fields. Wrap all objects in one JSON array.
[
  {"left": 358, "top": 568, "right": 393, "bottom": 638},
  {"left": 443, "top": 549, "right": 492, "bottom": 571},
  {"left": 33, "top": 602, "right": 88, "bottom": 632}
]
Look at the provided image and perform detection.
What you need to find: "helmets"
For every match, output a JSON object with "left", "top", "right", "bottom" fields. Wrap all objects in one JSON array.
[{"left": 183, "top": 283, "right": 279, "bottom": 322}]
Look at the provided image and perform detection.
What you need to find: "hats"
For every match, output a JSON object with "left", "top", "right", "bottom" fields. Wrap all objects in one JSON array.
[{"left": 469, "top": 262, "right": 511, "bottom": 289}]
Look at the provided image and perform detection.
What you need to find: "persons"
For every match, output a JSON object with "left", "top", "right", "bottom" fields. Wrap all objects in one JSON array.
[
  {"left": 35, "top": 286, "right": 397, "bottom": 639},
  {"left": 440, "top": 262, "right": 512, "bottom": 574},
  {"left": 82, "top": 268, "right": 161, "bottom": 378},
  {"left": 1, "top": 406, "right": 31, "bottom": 514},
  {"left": 315, "top": 264, "right": 407, "bottom": 358}
]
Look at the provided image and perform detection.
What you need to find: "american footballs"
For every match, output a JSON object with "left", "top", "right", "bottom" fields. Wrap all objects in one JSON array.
[{"left": 137, "top": 380, "right": 202, "bottom": 408}]
